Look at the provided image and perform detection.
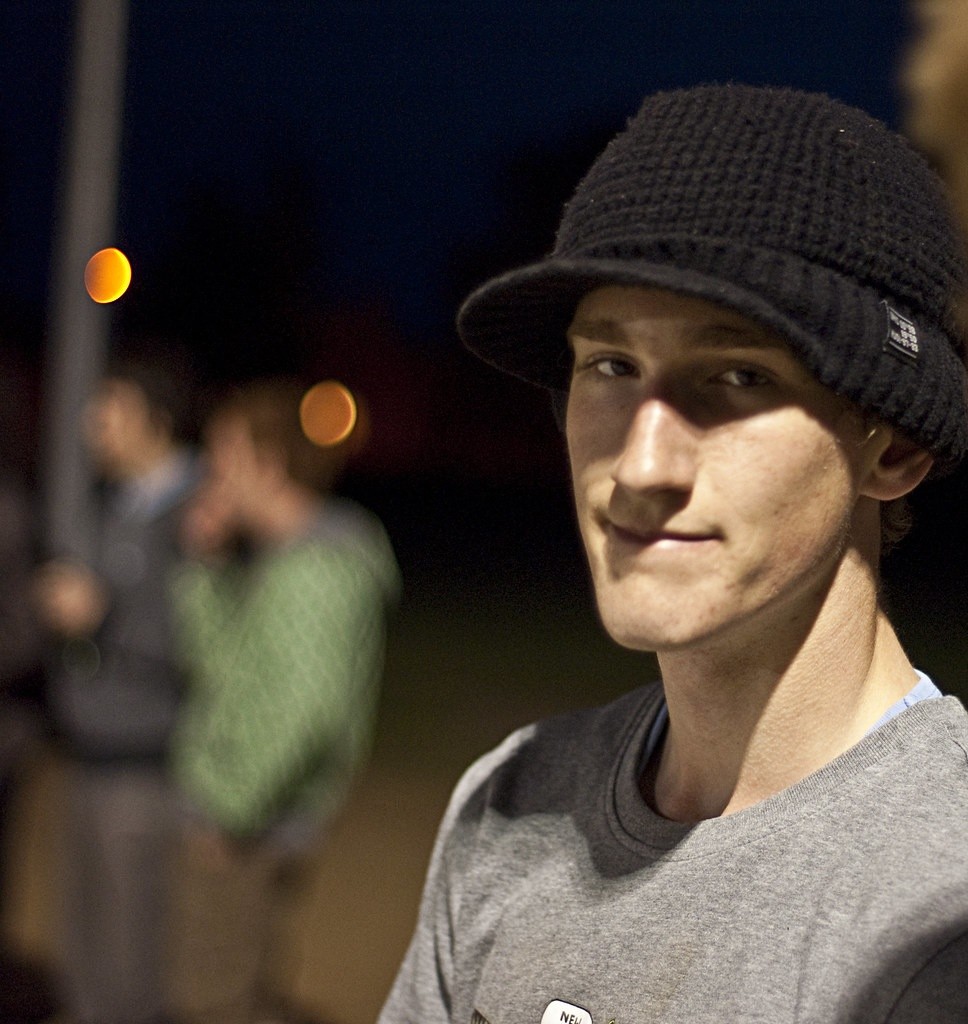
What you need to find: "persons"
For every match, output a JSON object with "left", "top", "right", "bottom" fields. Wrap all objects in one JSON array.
[
  {"left": 163, "top": 382, "right": 410, "bottom": 1024},
  {"left": 24, "top": 358, "right": 225, "bottom": 1018},
  {"left": 357, "top": 80, "right": 968, "bottom": 1022}
]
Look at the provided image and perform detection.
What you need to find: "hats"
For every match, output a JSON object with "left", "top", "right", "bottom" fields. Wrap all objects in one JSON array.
[{"left": 455, "top": 84, "right": 966, "bottom": 460}]
